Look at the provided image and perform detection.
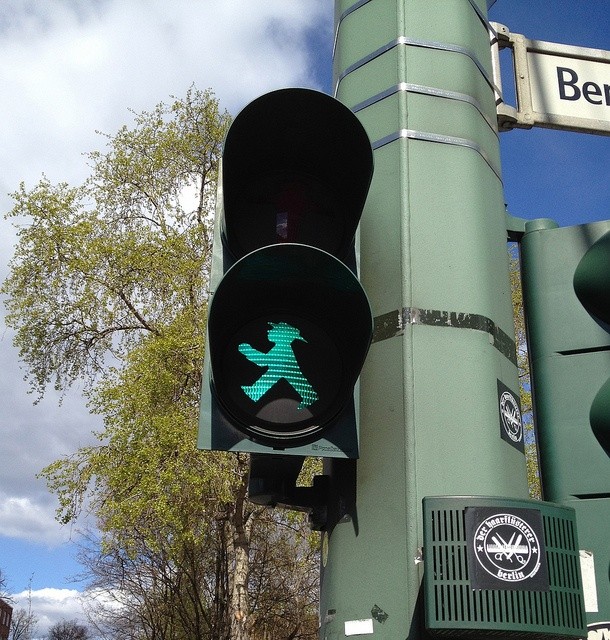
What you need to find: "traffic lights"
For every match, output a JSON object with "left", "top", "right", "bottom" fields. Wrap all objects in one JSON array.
[{"left": 215, "top": 294, "right": 357, "bottom": 447}]
